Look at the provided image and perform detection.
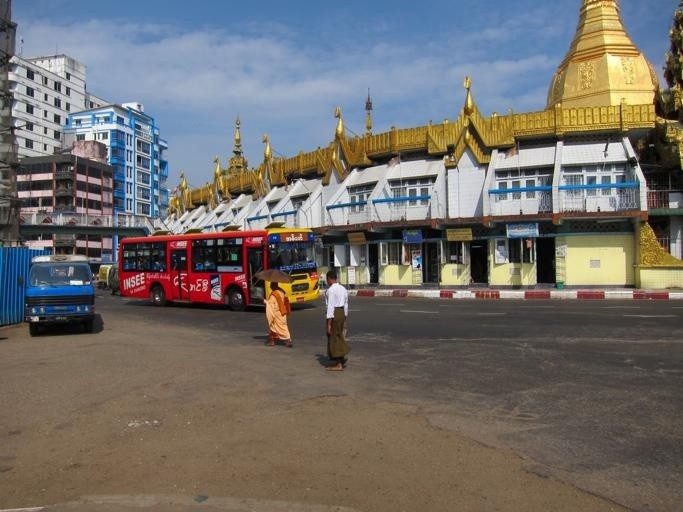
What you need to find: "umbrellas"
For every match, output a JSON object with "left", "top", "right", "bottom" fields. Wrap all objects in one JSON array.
[{"left": 252, "top": 268, "right": 295, "bottom": 284}]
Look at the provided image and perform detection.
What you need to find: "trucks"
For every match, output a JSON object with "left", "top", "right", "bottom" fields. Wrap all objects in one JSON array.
[{"left": 17, "top": 253, "right": 96, "bottom": 336}]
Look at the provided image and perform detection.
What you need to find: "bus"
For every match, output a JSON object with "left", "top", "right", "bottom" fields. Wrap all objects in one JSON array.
[{"left": 119, "top": 221, "right": 321, "bottom": 311}]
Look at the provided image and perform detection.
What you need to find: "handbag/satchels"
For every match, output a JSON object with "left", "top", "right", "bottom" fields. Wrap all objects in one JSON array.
[{"left": 284, "top": 297, "right": 291, "bottom": 315}]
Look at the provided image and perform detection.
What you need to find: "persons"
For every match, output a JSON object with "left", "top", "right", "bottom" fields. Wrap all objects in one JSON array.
[
  {"left": 255, "top": 281, "right": 292, "bottom": 348},
  {"left": 123, "top": 255, "right": 165, "bottom": 272},
  {"left": 322, "top": 271, "right": 351, "bottom": 371}
]
